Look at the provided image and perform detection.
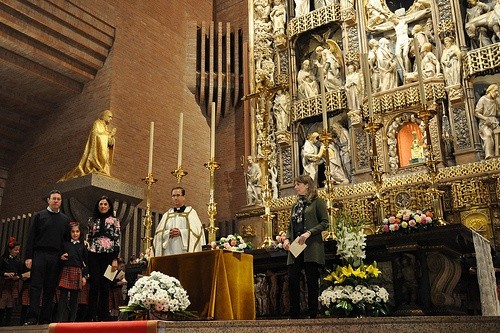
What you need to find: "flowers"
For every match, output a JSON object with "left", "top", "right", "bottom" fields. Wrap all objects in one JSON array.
[
  {"left": 119, "top": 271, "right": 200, "bottom": 321},
  {"left": 383, "top": 209, "right": 433, "bottom": 232},
  {"left": 130, "top": 248, "right": 150, "bottom": 266},
  {"left": 207, "top": 233, "right": 253, "bottom": 251},
  {"left": 318, "top": 209, "right": 390, "bottom": 318},
  {"left": 272, "top": 231, "right": 287, "bottom": 249},
  {"left": 9, "top": 234, "right": 15, "bottom": 247}
]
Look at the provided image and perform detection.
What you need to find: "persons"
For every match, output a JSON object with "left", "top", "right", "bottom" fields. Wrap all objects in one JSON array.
[
  {"left": 58, "top": 110, "right": 116, "bottom": 182},
  {"left": 0, "top": 189, "right": 90, "bottom": 326},
  {"left": 86, "top": 195, "right": 121, "bottom": 322},
  {"left": 153, "top": 187, "right": 206, "bottom": 257},
  {"left": 282, "top": 175, "right": 329, "bottom": 317},
  {"left": 243, "top": 0, "right": 500, "bottom": 206}
]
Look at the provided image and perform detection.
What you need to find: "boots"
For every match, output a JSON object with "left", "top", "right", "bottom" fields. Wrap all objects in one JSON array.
[
  {"left": 27, "top": 312, "right": 32, "bottom": 319},
  {"left": 69, "top": 305, "right": 78, "bottom": 322},
  {"left": 57, "top": 304, "right": 64, "bottom": 322},
  {"left": 21, "top": 311, "right": 27, "bottom": 324},
  {"left": 0, "top": 309, "right": 4, "bottom": 326},
  {"left": 5, "top": 308, "right": 13, "bottom": 325},
  {"left": 114, "top": 316, "right": 118, "bottom": 321}
]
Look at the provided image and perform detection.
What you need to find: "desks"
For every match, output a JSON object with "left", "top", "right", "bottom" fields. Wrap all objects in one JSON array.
[
  {"left": 146, "top": 249, "right": 256, "bottom": 321},
  {"left": 125, "top": 224, "right": 500, "bottom": 316}
]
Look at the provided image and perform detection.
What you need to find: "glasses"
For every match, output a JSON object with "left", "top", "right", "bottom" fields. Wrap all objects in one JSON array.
[{"left": 172, "top": 194, "right": 183, "bottom": 197}]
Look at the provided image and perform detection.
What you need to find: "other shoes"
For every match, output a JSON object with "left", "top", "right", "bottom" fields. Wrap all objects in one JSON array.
[
  {"left": 27, "top": 318, "right": 37, "bottom": 325},
  {"left": 100, "top": 313, "right": 114, "bottom": 320},
  {"left": 306, "top": 311, "right": 317, "bottom": 316},
  {"left": 288, "top": 308, "right": 300, "bottom": 317},
  {"left": 40, "top": 320, "right": 50, "bottom": 324},
  {"left": 89, "top": 314, "right": 99, "bottom": 322}
]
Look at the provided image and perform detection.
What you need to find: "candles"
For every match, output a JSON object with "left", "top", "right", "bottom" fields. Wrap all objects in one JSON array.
[
  {"left": 362, "top": 54, "right": 373, "bottom": 118},
  {"left": 210, "top": 101, "right": 215, "bottom": 159},
  {"left": 412, "top": 37, "right": 425, "bottom": 103},
  {"left": 318, "top": 66, "right": 327, "bottom": 132},
  {"left": 148, "top": 122, "right": 154, "bottom": 175},
  {"left": 177, "top": 113, "right": 183, "bottom": 169}
]
